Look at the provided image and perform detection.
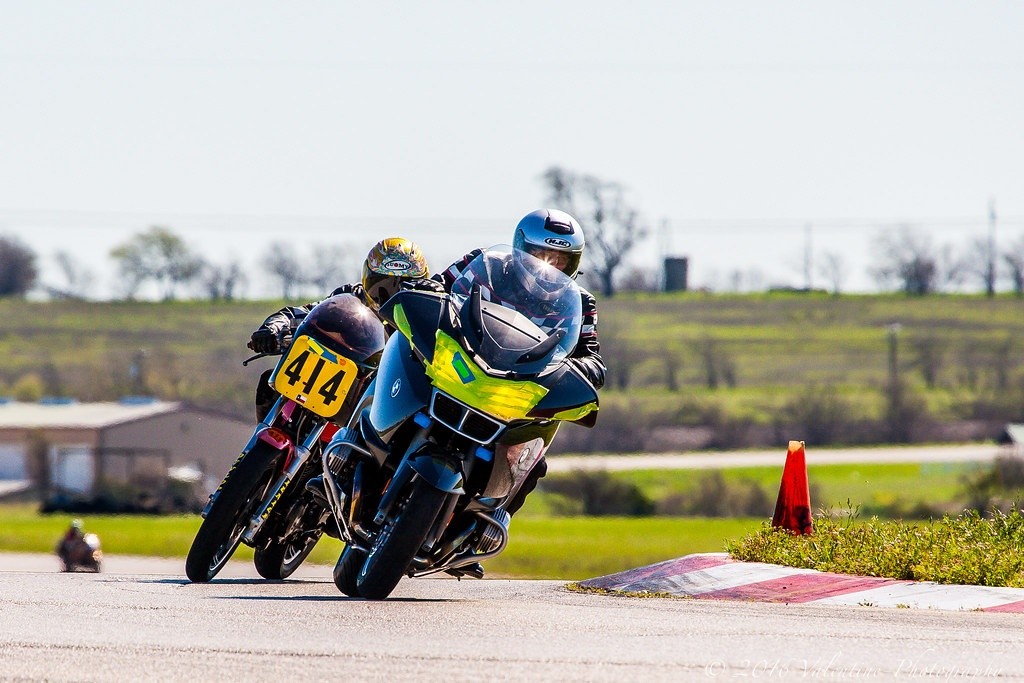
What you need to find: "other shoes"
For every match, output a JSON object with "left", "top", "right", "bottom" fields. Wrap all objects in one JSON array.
[
  {"left": 451, "top": 546, "right": 484, "bottom": 579},
  {"left": 306, "top": 476, "right": 328, "bottom": 501}
]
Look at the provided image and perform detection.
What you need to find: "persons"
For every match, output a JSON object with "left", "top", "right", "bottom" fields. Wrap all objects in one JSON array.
[
  {"left": 389, "top": 212, "right": 610, "bottom": 581},
  {"left": 245, "top": 239, "right": 435, "bottom": 436},
  {"left": 60, "top": 518, "right": 103, "bottom": 573}
]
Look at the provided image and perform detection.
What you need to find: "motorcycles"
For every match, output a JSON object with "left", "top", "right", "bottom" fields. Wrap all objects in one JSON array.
[
  {"left": 183, "top": 291, "right": 388, "bottom": 586},
  {"left": 320, "top": 246, "right": 599, "bottom": 600}
]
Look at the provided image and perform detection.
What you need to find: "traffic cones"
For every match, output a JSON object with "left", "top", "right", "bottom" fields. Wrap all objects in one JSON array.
[{"left": 771, "top": 438, "right": 811, "bottom": 540}]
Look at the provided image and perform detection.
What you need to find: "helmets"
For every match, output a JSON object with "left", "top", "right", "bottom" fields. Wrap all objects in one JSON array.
[
  {"left": 513, "top": 209, "right": 584, "bottom": 302},
  {"left": 361, "top": 238, "right": 429, "bottom": 312}
]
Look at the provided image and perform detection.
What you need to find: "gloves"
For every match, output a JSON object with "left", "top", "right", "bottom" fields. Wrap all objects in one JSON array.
[{"left": 250, "top": 330, "right": 281, "bottom": 355}]
